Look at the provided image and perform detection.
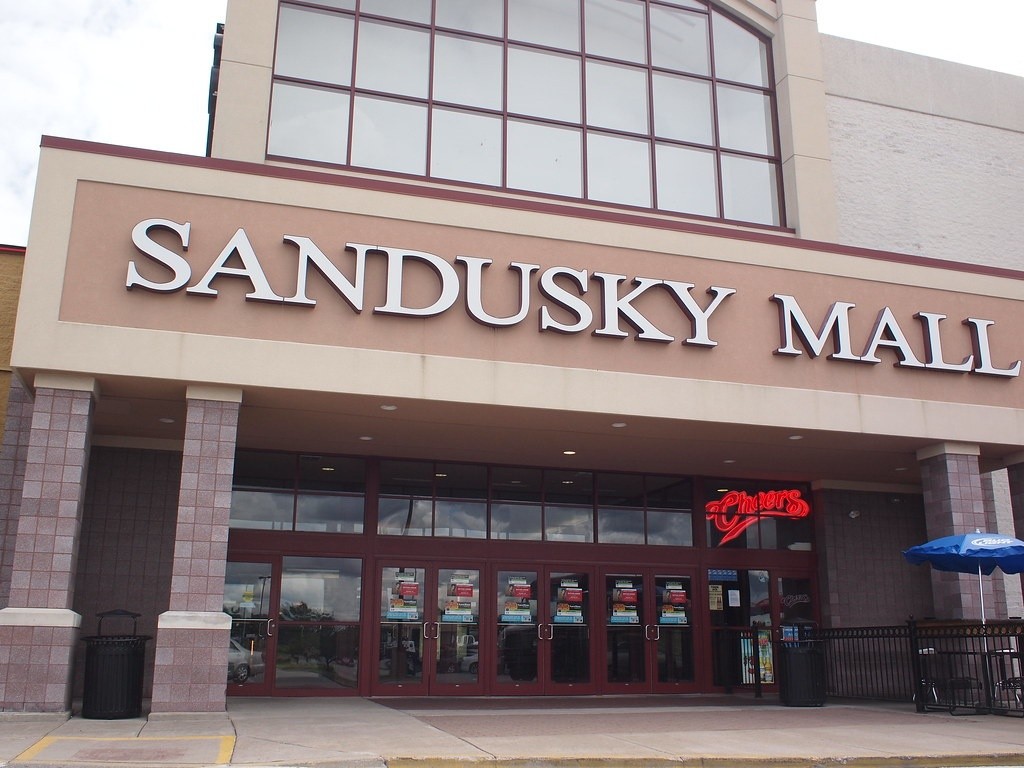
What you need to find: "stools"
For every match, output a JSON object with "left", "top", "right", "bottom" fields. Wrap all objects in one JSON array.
[
  {"left": 990, "top": 648, "right": 1022, "bottom": 709},
  {"left": 947, "top": 677, "right": 983, "bottom": 710},
  {"left": 912, "top": 648, "right": 938, "bottom": 705}
]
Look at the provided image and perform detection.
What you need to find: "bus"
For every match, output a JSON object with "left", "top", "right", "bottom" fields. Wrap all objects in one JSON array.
[{"left": 495, "top": 575, "right": 740, "bottom": 686}]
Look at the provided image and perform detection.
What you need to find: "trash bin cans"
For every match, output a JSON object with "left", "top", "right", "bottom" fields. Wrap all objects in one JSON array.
[
  {"left": 81, "top": 610, "right": 153, "bottom": 720},
  {"left": 775, "top": 618, "right": 827, "bottom": 707}
]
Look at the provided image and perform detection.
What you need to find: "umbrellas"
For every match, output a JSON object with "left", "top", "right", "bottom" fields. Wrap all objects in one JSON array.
[{"left": 901, "top": 528, "right": 1024, "bottom": 713}]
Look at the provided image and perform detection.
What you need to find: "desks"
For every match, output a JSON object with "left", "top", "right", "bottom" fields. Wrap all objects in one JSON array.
[{"left": 905, "top": 618, "right": 1024, "bottom": 713}]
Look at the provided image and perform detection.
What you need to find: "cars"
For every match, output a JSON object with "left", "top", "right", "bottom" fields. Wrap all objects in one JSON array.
[
  {"left": 334, "top": 645, "right": 391, "bottom": 682},
  {"left": 459, "top": 653, "right": 479, "bottom": 675},
  {"left": 227, "top": 639, "right": 266, "bottom": 685}
]
